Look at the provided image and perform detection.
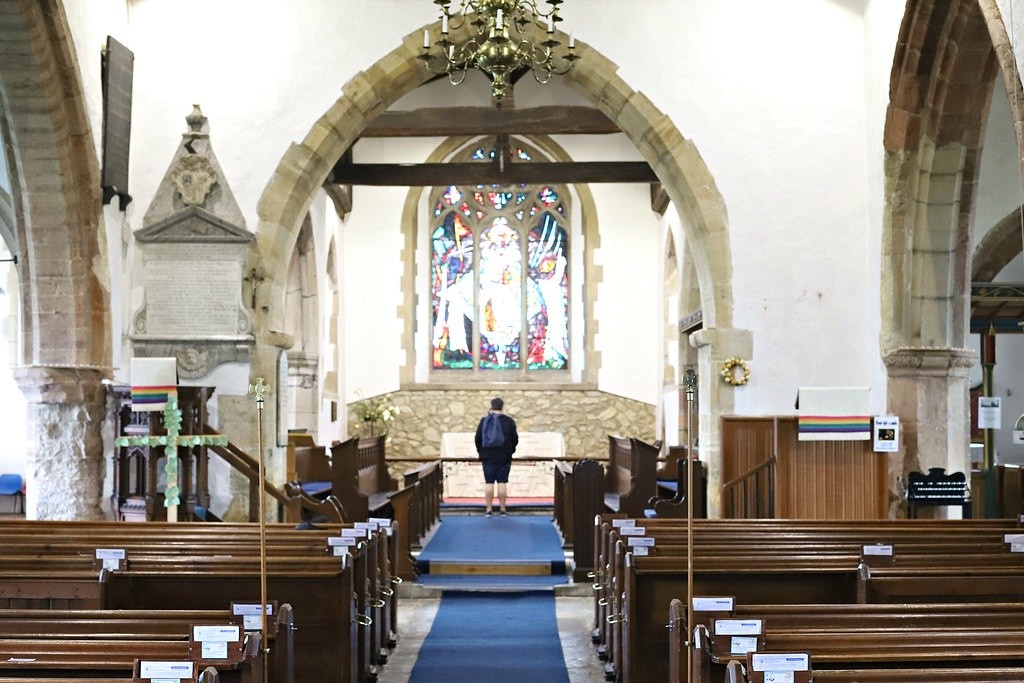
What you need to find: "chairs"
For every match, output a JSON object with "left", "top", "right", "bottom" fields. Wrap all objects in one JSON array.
[{"left": 0, "top": 474, "right": 23, "bottom": 514}]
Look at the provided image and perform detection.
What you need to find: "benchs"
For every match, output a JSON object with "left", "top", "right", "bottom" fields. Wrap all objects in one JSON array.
[
  {"left": 588, "top": 433, "right": 1024, "bottom": 683},
  {"left": 0, "top": 434, "right": 399, "bottom": 683}
]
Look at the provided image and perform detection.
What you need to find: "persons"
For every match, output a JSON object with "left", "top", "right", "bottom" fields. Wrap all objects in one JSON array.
[{"left": 475, "top": 397, "right": 517, "bottom": 518}]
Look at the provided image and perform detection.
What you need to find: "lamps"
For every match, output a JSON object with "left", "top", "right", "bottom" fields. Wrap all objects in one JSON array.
[{"left": 416, "top": 0, "right": 581, "bottom": 111}]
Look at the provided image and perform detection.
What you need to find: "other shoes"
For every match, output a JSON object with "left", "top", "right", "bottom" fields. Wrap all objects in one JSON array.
[
  {"left": 500, "top": 510, "right": 508, "bottom": 518},
  {"left": 485, "top": 510, "right": 492, "bottom": 518}
]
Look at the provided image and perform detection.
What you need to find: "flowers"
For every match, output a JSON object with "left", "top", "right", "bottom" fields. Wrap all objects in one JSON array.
[{"left": 351, "top": 387, "right": 399, "bottom": 436}]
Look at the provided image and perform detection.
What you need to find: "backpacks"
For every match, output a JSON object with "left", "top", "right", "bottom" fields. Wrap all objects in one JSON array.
[{"left": 482, "top": 413, "right": 506, "bottom": 447}]
